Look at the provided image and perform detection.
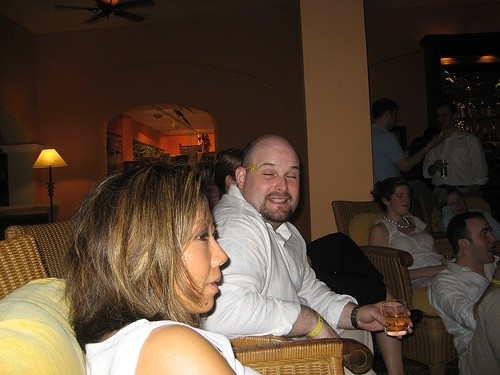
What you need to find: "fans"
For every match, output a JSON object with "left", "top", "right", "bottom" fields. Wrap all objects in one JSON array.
[{"left": 52, "top": 0, "right": 158, "bottom": 26}]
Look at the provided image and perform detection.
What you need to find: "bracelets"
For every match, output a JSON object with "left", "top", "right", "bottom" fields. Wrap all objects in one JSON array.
[
  {"left": 351, "top": 306, "right": 365, "bottom": 331},
  {"left": 306, "top": 310, "right": 323, "bottom": 337},
  {"left": 490, "top": 278, "right": 499, "bottom": 286}
]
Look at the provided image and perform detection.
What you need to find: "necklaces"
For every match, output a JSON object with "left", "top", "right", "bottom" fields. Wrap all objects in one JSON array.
[{"left": 383, "top": 216, "right": 410, "bottom": 229}]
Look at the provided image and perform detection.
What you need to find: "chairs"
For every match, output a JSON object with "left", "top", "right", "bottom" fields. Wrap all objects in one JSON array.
[
  {"left": 330, "top": 201, "right": 487, "bottom": 375},
  {"left": 1, "top": 219, "right": 375, "bottom": 375}
]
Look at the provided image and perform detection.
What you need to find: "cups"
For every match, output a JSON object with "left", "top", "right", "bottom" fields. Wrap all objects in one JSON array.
[
  {"left": 441, "top": 159, "right": 447, "bottom": 177},
  {"left": 383, "top": 299, "right": 409, "bottom": 336}
]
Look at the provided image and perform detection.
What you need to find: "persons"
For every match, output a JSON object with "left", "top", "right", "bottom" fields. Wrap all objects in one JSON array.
[
  {"left": 70, "top": 133, "right": 413, "bottom": 375},
  {"left": 368, "top": 98, "right": 500, "bottom": 375}
]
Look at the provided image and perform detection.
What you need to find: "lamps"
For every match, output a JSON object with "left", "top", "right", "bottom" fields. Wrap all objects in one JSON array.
[{"left": 32, "top": 146, "right": 69, "bottom": 222}]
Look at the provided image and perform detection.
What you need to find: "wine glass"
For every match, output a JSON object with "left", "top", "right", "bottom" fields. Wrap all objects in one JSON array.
[{"left": 441, "top": 245, "right": 452, "bottom": 267}]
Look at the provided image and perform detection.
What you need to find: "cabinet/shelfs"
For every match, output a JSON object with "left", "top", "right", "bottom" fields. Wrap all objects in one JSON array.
[{"left": 418, "top": 30, "right": 500, "bottom": 164}]
